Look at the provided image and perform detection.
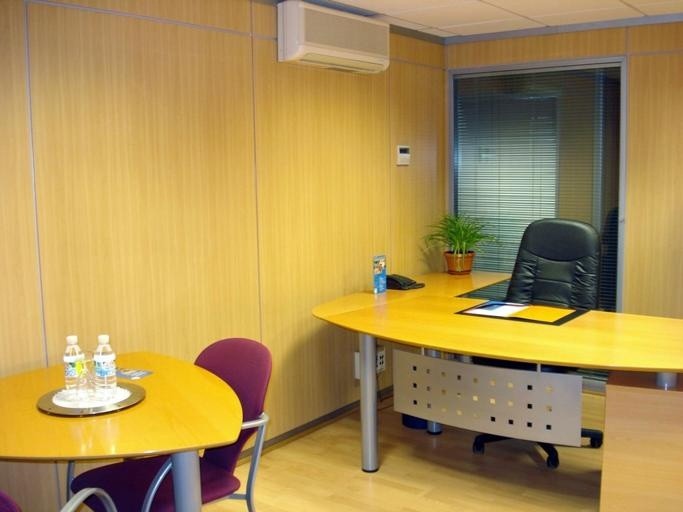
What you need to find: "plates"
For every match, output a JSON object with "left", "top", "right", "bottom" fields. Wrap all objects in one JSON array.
[{"left": 36, "top": 379, "right": 146, "bottom": 418}]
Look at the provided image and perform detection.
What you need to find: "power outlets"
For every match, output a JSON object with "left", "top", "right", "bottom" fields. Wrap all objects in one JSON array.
[{"left": 354, "top": 345, "right": 386, "bottom": 382}]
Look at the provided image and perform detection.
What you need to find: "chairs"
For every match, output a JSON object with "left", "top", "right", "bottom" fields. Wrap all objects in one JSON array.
[
  {"left": 0, "top": 487, "right": 116, "bottom": 512},
  {"left": 73, "top": 335, "right": 272, "bottom": 512},
  {"left": 472, "top": 216, "right": 604, "bottom": 467}
]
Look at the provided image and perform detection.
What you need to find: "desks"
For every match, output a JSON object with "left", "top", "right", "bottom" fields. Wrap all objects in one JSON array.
[
  {"left": 0, "top": 349, "right": 239, "bottom": 512},
  {"left": 311, "top": 270, "right": 683, "bottom": 512}
]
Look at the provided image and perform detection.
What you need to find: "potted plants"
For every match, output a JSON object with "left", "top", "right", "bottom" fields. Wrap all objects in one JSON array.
[{"left": 421, "top": 211, "right": 500, "bottom": 276}]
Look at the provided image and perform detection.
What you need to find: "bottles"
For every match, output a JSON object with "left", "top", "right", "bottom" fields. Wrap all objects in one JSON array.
[
  {"left": 63, "top": 335, "right": 89, "bottom": 401},
  {"left": 91, "top": 334, "right": 117, "bottom": 401}
]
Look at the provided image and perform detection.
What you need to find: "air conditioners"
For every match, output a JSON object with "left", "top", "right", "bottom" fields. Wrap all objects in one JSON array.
[{"left": 277, "top": 0, "right": 392, "bottom": 75}]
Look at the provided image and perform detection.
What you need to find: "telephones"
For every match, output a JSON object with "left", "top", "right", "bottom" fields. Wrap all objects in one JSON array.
[{"left": 386, "top": 274, "right": 416, "bottom": 289}]
